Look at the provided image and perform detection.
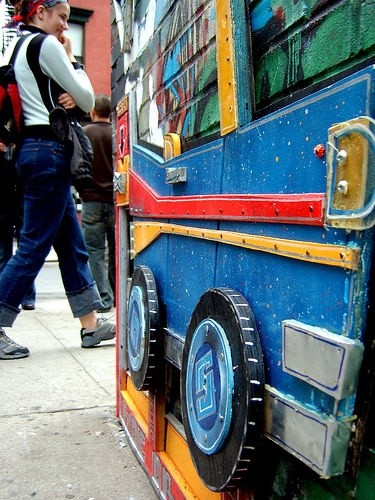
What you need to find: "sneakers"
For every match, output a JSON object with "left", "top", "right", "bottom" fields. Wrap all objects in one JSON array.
[
  {"left": 0, "top": 327, "right": 30, "bottom": 360},
  {"left": 79, "top": 318, "right": 117, "bottom": 347}
]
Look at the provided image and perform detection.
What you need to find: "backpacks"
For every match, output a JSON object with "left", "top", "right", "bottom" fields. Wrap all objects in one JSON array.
[{"left": 0, "top": 33, "right": 32, "bottom": 165}]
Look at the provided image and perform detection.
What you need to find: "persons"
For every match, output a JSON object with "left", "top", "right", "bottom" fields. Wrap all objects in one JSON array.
[
  {"left": 0, "top": 0, "right": 117, "bottom": 360},
  {"left": 82, "top": 97, "right": 117, "bottom": 312}
]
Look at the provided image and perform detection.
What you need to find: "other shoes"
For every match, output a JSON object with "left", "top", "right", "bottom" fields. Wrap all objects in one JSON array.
[{"left": 22, "top": 303, "right": 35, "bottom": 311}]
